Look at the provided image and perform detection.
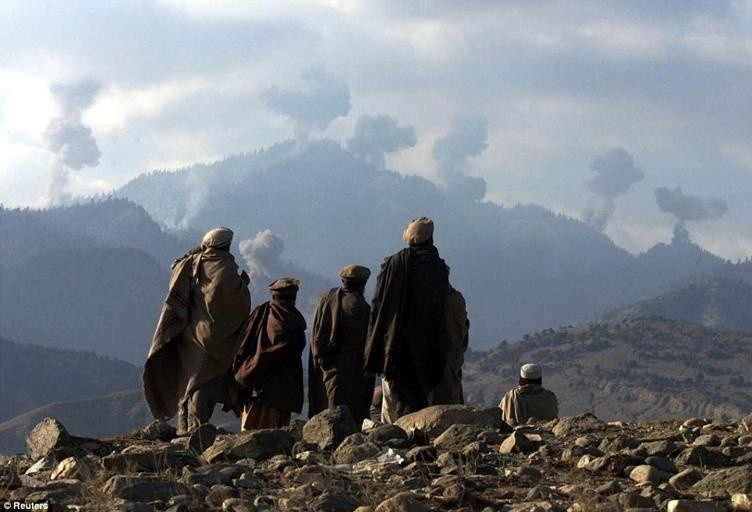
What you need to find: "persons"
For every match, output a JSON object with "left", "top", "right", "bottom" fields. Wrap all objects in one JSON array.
[
  {"left": 142, "top": 227, "right": 251, "bottom": 435},
  {"left": 500, "top": 363, "right": 558, "bottom": 428},
  {"left": 307, "top": 264, "right": 374, "bottom": 424},
  {"left": 229, "top": 277, "right": 307, "bottom": 432},
  {"left": 430, "top": 257, "right": 470, "bottom": 406},
  {"left": 362, "top": 217, "right": 455, "bottom": 423}
]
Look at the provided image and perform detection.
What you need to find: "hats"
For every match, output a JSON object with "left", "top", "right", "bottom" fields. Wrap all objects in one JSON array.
[
  {"left": 200, "top": 227, "right": 234, "bottom": 249},
  {"left": 403, "top": 216, "right": 434, "bottom": 244},
  {"left": 520, "top": 363, "right": 543, "bottom": 380},
  {"left": 268, "top": 277, "right": 300, "bottom": 292},
  {"left": 339, "top": 264, "right": 371, "bottom": 280}
]
